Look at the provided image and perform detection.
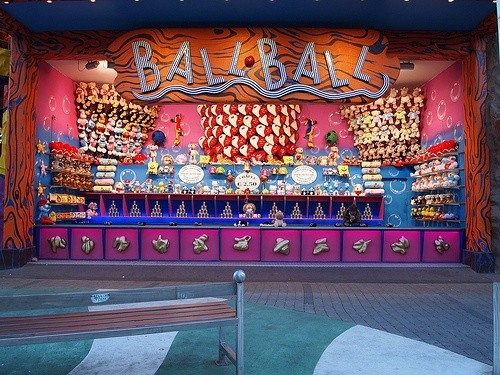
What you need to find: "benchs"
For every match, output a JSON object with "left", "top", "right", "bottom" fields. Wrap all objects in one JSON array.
[{"left": 0, "top": 268, "right": 244, "bottom": 375}]
